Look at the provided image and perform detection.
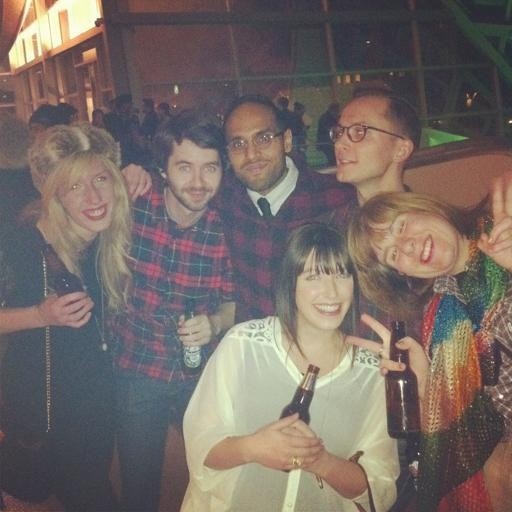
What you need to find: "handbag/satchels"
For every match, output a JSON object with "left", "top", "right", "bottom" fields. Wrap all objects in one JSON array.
[{"left": 0, "top": 422, "right": 67, "bottom": 504}]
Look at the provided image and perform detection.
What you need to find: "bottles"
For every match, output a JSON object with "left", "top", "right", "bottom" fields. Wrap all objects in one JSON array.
[
  {"left": 183, "top": 298, "right": 203, "bottom": 375},
  {"left": 386, "top": 321, "right": 420, "bottom": 438},
  {"left": 404, "top": 439, "right": 420, "bottom": 496},
  {"left": 43, "top": 243, "right": 102, "bottom": 351},
  {"left": 280, "top": 364, "right": 320, "bottom": 473}
]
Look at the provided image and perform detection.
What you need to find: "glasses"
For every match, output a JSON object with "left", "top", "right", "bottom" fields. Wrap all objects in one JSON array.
[
  {"left": 226, "top": 132, "right": 281, "bottom": 150},
  {"left": 328, "top": 123, "right": 405, "bottom": 143}
]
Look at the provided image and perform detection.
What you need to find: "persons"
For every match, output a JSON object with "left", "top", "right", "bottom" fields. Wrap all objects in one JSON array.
[{"left": 1, "top": 78, "right": 510, "bottom": 512}]
